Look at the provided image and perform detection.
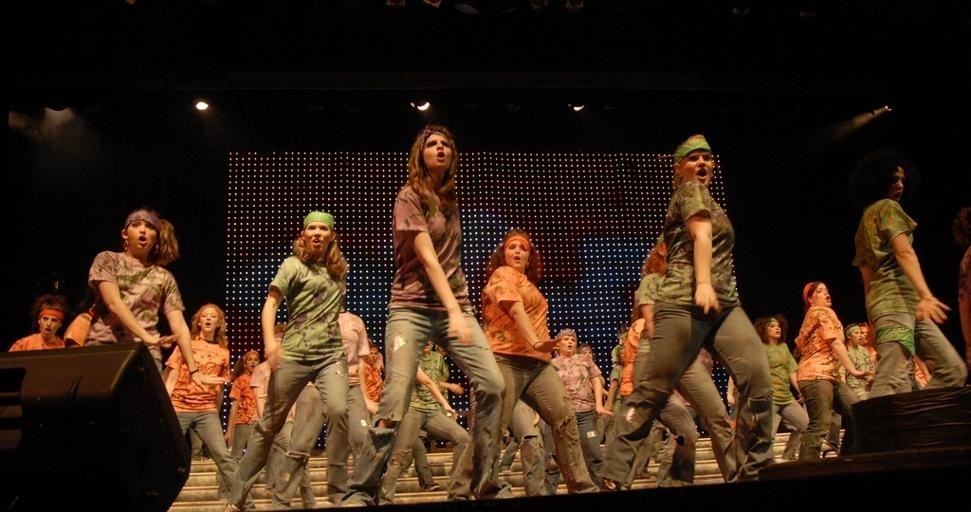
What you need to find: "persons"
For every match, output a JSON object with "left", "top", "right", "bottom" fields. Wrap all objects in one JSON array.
[
  {"left": 601, "top": 135, "right": 770, "bottom": 491},
  {"left": 855, "top": 156, "right": 967, "bottom": 400},
  {"left": 336, "top": 126, "right": 514, "bottom": 505},
  {"left": 955, "top": 207, "right": 971, "bottom": 376},
  {"left": 5, "top": 210, "right": 933, "bottom": 512}
]
